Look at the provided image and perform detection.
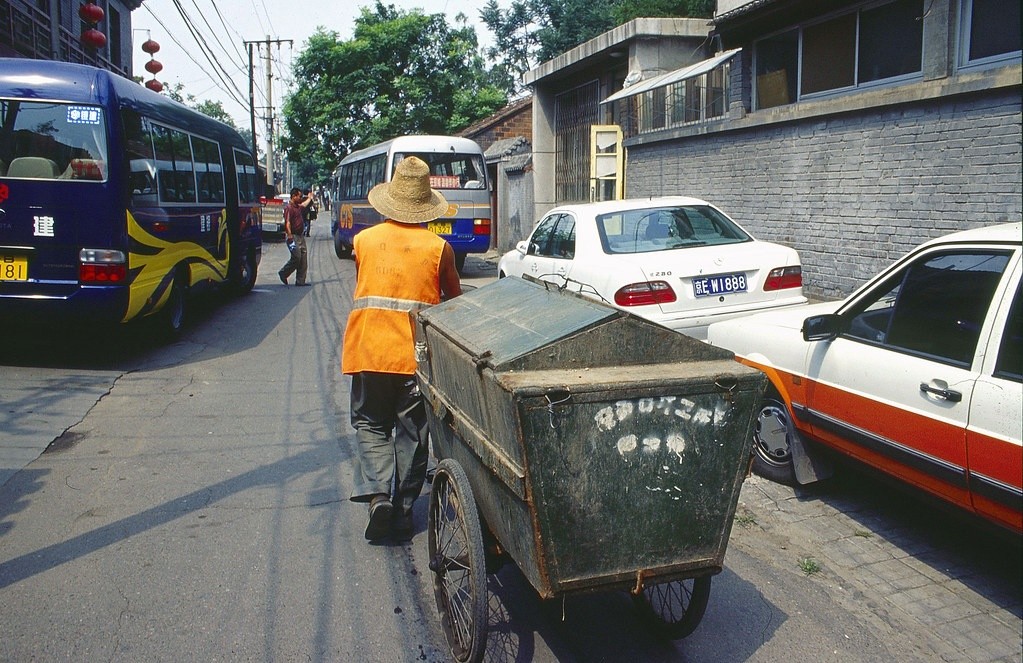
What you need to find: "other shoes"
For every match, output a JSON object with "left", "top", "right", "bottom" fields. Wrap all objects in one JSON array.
[
  {"left": 296, "top": 283, "right": 311, "bottom": 286},
  {"left": 395, "top": 525, "right": 415, "bottom": 542},
  {"left": 278, "top": 272, "right": 288, "bottom": 286},
  {"left": 364, "top": 501, "right": 393, "bottom": 540}
]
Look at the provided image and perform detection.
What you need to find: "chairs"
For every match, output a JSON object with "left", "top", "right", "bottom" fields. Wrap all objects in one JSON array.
[
  {"left": 646, "top": 222, "right": 671, "bottom": 239},
  {"left": 61, "top": 156, "right": 105, "bottom": 179},
  {"left": 464, "top": 180, "right": 483, "bottom": 188},
  {"left": 7, "top": 155, "right": 58, "bottom": 177}
]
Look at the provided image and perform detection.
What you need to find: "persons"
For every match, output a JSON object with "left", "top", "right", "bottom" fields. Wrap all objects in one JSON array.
[
  {"left": 339, "top": 156, "right": 463, "bottom": 549},
  {"left": 278, "top": 187, "right": 311, "bottom": 286},
  {"left": 300, "top": 190, "right": 310, "bottom": 237},
  {"left": 310, "top": 188, "right": 330, "bottom": 220}
]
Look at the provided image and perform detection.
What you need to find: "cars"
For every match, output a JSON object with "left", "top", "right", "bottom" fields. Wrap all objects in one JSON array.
[
  {"left": 261, "top": 193, "right": 291, "bottom": 240},
  {"left": 496, "top": 194, "right": 810, "bottom": 343},
  {"left": 702, "top": 221, "right": 1022, "bottom": 555}
]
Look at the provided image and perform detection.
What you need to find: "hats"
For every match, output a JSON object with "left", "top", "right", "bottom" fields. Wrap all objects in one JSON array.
[{"left": 367, "top": 156, "right": 450, "bottom": 223}]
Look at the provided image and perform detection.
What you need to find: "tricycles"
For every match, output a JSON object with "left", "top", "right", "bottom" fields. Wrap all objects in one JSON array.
[{"left": 394, "top": 271, "right": 771, "bottom": 663}]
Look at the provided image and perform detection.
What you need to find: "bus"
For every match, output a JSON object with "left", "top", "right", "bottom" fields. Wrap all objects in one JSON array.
[
  {"left": 0, "top": 56, "right": 264, "bottom": 358},
  {"left": 319, "top": 134, "right": 492, "bottom": 275}
]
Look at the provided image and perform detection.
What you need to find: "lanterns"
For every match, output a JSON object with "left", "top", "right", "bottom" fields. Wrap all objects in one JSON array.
[
  {"left": 145, "top": 60, "right": 163, "bottom": 74},
  {"left": 79, "top": 4, "right": 104, "bottom": 25},
  {"left": 142, "top": 40, "right": 160, "bottom": 54},
  {"left": 79, "top": 29, "right": 107, "bottom": 49},
  {"left": 145, "top": 79, "right": 163, "bottom": 93}
]
